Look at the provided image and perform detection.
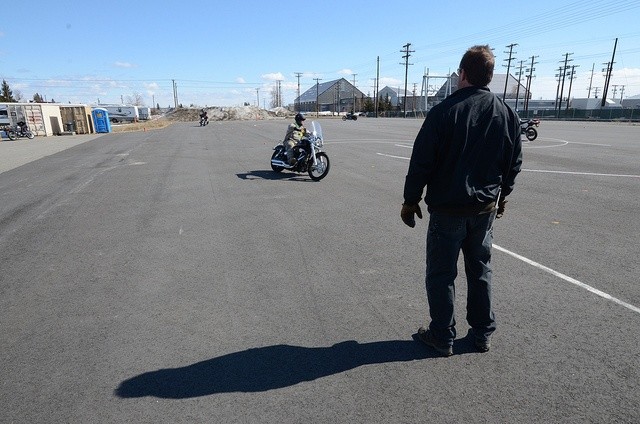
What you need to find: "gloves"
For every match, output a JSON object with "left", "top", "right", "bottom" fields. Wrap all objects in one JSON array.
[
  {"left": 400, "top": 198, "right": 422, "bottom": 227},
  {"left": 495, "top": 199, "right": 508, "bottom": 219}
]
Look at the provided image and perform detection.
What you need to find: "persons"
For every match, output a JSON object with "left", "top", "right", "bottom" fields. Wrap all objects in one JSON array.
[
  {"left": 400, "top": 44, "right": 523, "bottom": 356},
  {"left": 283, "top": 114, "right": 311, "bottom": 165}
]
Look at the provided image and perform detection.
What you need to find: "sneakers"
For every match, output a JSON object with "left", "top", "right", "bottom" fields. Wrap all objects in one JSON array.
[
  {"left": 287, "top": 157, "right": 296, "bottom": 165},
  {"left": 451, "top": 329, "right": 491, "bottom": 355},
  {"left": 418, "top": 325, "right": 454, "bottom": 357}
]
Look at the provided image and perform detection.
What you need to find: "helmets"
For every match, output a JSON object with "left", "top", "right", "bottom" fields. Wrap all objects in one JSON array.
[{"left": 295, "top": 113, "right": 306, "bottom": 121}]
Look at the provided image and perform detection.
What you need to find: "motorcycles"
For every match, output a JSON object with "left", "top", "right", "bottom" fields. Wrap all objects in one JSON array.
[
  {"left": 5, "top": 122, "right": 34, "bottom": 140},
  {"left": 270, "top": 121, "right": 329, "bottom": 181},
  {"left": 199, "top": 117, "right": 209, "bottom": 127},
  {"left": 515, "top": 109, "right": 540, "bottom": 141},
  {"left": 342, "top": 115, "right": 358, "bottom": 121}
]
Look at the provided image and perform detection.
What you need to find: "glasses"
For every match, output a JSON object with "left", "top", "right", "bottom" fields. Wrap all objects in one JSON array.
[{"left": 457, "top": 68, "right": 463, "bottom": 75}]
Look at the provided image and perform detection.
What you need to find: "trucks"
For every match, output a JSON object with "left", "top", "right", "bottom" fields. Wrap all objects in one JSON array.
[{"left": 92, "top": 107, "right": 139, "bottom": 123}]
